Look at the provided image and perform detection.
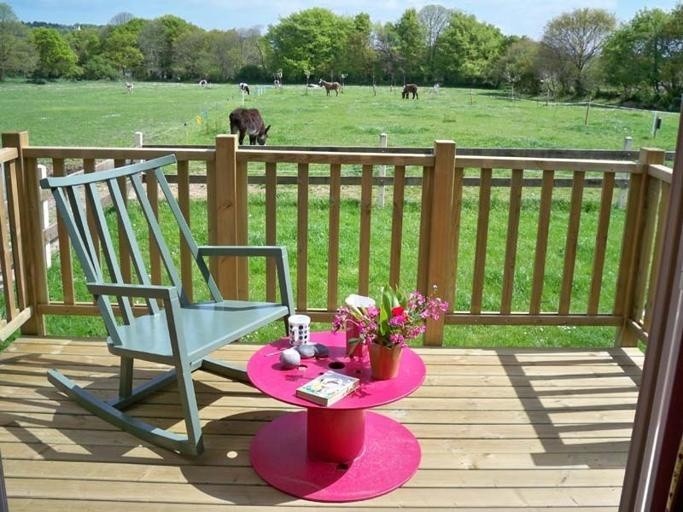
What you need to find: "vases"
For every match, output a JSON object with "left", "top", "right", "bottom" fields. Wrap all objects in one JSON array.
[{"left": 369, "top": 335, "right": 404, "bottom": 380}]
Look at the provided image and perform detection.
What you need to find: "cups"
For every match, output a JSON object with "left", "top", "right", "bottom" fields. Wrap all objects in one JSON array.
[
  {"left": 287, "top": 314, "right": 311, "bottom": 347},
  {"left": 344, "top": 293, "right": 376, "bottom": 358}
]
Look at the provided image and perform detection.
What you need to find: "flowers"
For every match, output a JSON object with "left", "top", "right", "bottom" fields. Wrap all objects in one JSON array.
[{"left": 331, "top": 283, "right": 449, "bottom": 357}]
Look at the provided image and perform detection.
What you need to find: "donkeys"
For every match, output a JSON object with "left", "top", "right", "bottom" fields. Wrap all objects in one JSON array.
[
  {"left": 229, "top": 107, "right": 271, "bottom": 145},
  {"left": 401, "top": 84, "right": 419, "bottom": 99},
  {"left": 320, "top": 80, "right": 341, "bottom": 97}
]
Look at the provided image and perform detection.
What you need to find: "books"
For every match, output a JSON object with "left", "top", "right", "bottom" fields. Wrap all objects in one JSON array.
[{"left": 294, "top": 369, "right": 361, "bottom": 408}]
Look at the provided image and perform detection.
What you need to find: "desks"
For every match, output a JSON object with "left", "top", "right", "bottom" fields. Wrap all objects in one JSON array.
[{"left": 247, "top": 327, "right": 427, "bottom": 502}]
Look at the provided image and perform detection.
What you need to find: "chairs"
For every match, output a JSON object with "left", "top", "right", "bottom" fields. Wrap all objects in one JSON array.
[{"left": 38, "top": 152, "right": 295, "bottom": 459}]
